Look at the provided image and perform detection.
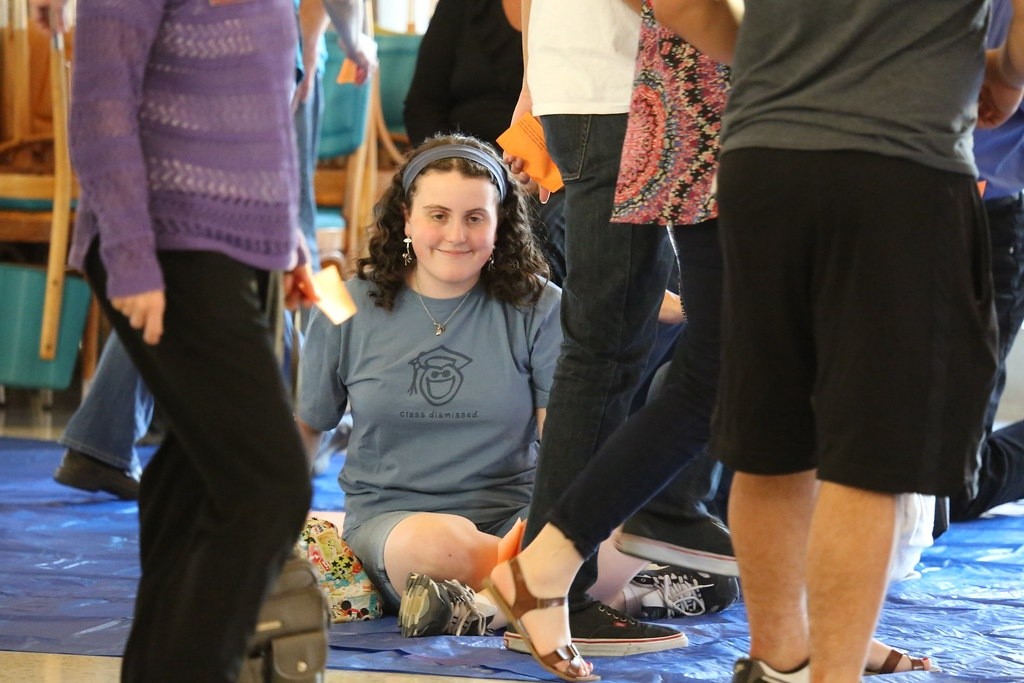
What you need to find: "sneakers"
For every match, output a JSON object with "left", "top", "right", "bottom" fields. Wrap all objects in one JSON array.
[
  {"left": 630, "top": 565, "right": 738, "bottom": 620},
  {"left": 503, "top": 599, "right": 689, "bottom": 655},
  {"left": 396, "top": 573, "right": 497, "bottom": 640},
  {"left": 613, "top": 509, "right": 750, "bottom": 577},
  {"left": 730, "top": 655, "right": 810, "bottom": 683},
  {"left": 54, "top": 448, "right": 140, "bottom": 499}
]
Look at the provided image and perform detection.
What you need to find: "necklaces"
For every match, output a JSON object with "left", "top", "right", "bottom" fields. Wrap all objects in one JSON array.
[{"left": 410, "top": 264, "right": 472, "bottom": 336}]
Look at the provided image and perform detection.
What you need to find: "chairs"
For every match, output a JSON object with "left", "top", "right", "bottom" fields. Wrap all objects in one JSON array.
[{"left": 0, "top": 1, "right": 425, "bottom": 410}]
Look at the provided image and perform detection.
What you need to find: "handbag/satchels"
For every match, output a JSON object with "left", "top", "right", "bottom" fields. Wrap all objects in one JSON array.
[
  {"left": 295, "top": 517, "right": 383, "bottom": 623},
  {"left": 236, "top": 558, "right": 332, "bottom": 682}
]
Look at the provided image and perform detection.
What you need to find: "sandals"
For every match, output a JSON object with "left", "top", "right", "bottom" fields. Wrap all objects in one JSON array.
[
  {"left": 863, "top": 649, "right": 942, "bottom": 676},
  {"left": 481, "top": 558, "right": 601, "bottom": 682}
]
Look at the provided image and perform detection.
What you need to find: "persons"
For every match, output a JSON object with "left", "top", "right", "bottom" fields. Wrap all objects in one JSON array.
[{"left": 53, "top": 0, "right": 1024, "bottom": 683}]
